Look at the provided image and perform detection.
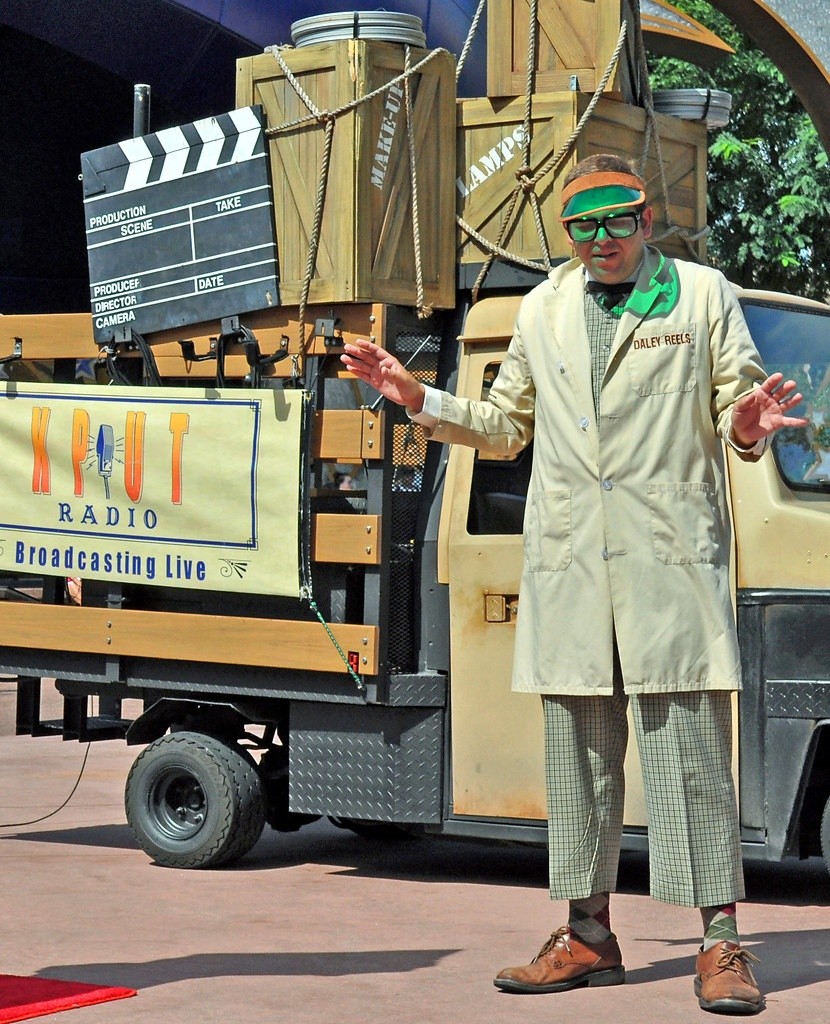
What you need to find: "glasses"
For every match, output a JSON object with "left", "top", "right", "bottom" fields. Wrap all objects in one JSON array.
[{"left": 567, "top": 209, "right": 641, "bottom": 242}]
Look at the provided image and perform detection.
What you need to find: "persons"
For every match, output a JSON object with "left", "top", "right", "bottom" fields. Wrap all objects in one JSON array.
[{"left": 342, "top": 153, "right": 810, "bottom": 1015}]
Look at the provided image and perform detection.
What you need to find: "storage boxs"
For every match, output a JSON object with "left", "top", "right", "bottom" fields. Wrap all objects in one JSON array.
[
  {"left": 231, "top": 51, "right": 457, "bottom": 308},
  {"left": 451, "top": 96, "right": 711, "bottom": 270},
  {"left": 488, "top": 0, "right": 636, "bottom": 103}
]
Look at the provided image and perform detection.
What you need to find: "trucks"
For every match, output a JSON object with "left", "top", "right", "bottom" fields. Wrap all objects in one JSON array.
[{"left": 0, "top": 1, "right": 829, "bottom": 898}]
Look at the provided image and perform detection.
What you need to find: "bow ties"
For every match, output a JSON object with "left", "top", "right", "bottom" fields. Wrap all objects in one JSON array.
[{"left": 584, "top": 280, "right": 635, "bottom": 311}]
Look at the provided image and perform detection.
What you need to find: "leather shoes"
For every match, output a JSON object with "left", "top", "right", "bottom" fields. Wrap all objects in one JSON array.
[
  {"left": 693, "top": 941, "right": 763, "bottom": 1011},
  {"left": 493, "top": 926, "right": 626, "bottom": 993}
]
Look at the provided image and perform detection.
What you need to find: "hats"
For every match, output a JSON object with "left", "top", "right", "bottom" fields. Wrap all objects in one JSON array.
[{"left": 559, "top": 172, "right": 645, "bottom": 222}]
